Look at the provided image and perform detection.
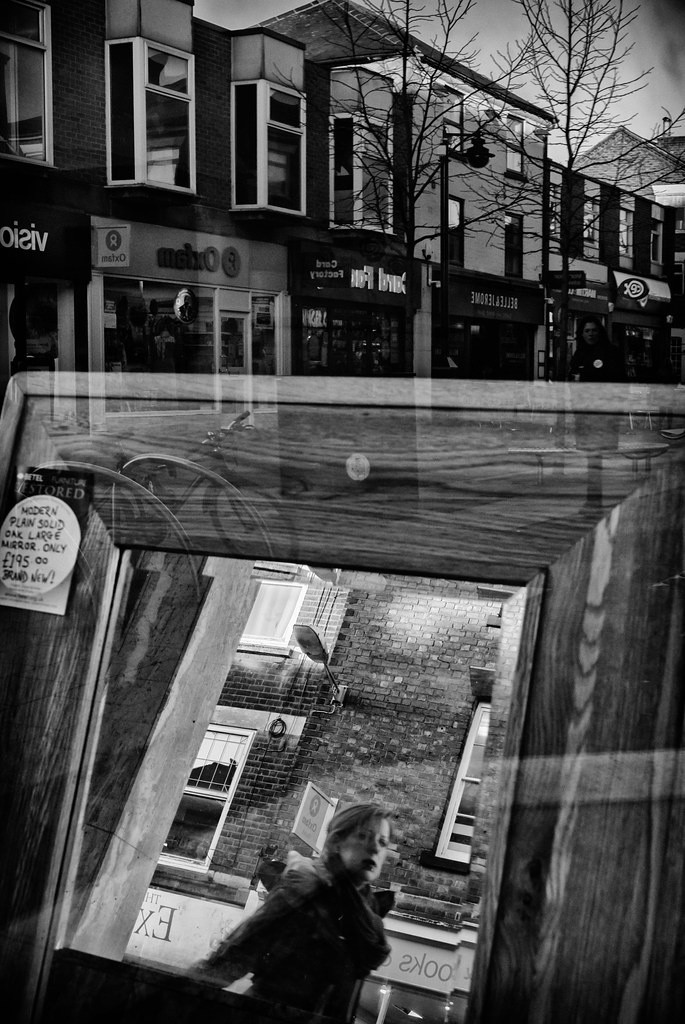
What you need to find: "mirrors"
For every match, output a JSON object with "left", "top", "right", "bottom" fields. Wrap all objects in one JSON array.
[{"left": 0, "top": 371, "right": 685, "bottom": 1024}]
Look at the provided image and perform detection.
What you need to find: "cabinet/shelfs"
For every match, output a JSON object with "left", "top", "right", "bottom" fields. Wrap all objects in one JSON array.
[{"left": 183, "top": 331, "right": 232, "bottom": 359}]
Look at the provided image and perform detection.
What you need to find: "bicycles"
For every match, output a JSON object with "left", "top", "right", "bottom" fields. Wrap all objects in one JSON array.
[{"left": 87, "top": 409, "right": 297, "bottom": 564}]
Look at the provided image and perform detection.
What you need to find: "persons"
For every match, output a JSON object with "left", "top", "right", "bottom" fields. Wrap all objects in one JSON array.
[
  {"left": 566, "top": 314, "right": 626, "bottom": 382},
  {"left": 150, "top": 328, "right": 180, "bottom": 374},
  {"left": 186, "top": 804, "right": 390, "bottom": 1024}
]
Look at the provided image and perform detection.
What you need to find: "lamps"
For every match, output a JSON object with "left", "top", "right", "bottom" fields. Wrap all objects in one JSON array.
[
  {"left": 466, "top": 137, "right": 496, "bottom": 168},
  {"left": 291, "top": 623, "right": 348, "bottom": 706}
]
